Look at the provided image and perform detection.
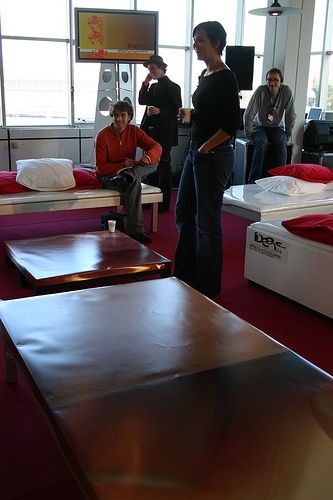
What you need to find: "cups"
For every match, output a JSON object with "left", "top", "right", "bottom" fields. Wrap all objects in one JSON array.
[
  {"left": 178, "top": 107, "right": 192, "bottom": 124},
  {"left": 108, "top": 220, "right": 116, "bottom": 232}
]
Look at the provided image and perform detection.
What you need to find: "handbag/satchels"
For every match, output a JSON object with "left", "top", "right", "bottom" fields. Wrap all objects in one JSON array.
[{"left": 101, "top": 210, "right": 127, "bottom": 233}]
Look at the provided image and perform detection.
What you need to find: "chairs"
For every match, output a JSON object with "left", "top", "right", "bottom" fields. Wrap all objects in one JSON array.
[{"left": 302, "top": 119, "right": 333, "bottom": 167}]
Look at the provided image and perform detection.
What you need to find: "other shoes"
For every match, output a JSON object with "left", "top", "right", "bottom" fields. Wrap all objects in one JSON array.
[{"left": 130, "top": 232, "right": 152, "bottom": 243}]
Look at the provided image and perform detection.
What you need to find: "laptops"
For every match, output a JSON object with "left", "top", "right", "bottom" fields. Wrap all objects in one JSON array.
[{"left": 305, "top": 108, "right": 323, "bottom": 124}]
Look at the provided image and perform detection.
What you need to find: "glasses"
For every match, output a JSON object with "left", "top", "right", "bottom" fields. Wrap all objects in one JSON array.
[{"left": 266, "top": 78, "right": 281, "bottom": 83}]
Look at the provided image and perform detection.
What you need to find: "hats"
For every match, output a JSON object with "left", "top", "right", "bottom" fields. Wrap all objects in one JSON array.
[{"left": 143, "top": 56, "right": 168, "bottom": 68}]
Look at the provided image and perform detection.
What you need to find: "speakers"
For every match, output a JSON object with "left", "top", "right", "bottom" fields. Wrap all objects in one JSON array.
[
  {"left": 233, "top": 138, "right": 294, "bottom": 185},
  {"left": 302, "top": 119, "right": 333, "bottom": 153},
  {"left": 225, "top": 46, "right": 255, "bottom": 90}
]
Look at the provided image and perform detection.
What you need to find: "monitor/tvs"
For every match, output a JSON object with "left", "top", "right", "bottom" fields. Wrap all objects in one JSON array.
[{"left": 74, "top": 8, "right": 159, "bottom": 64}]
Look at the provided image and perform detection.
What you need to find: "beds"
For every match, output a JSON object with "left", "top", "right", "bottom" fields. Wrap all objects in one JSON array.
[{"left": 0, "top": 157, "right": 333, "bottom": 320}]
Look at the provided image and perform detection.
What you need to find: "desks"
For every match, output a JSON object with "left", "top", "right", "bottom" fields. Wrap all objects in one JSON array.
[
  {"left": 4, "top": 229, "right": 173, "bottom": 296},
  {"left": 0, "top": 276, "right": 333, "bottom": 500}
]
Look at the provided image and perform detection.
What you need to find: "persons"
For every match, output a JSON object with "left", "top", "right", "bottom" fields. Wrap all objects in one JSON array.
[
  {"left": 95, "top": 101, "right": 162, "bottom": 244},
  {"left": 243, "top": 68, "right": 298, "bottom": 184},
  {"left": 173, "top": 21, "right": 241, "bottom": 301},
  {"left": 138, "top": 55, "right": 182, "bottom": 214}
]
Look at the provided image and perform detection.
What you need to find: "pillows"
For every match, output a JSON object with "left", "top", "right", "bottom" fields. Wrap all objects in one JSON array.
[
  {"left": 15, "top": 158, "right": 76, "bottom": 192},
  {"left": 268, "top": 164, "right": 333, "bottom": 184},
  {"left": 254, "top": 175, "right": 329, "bottom": 197},
  {"left": 281, "top": 213, "right": 333, "bottom": 245}
]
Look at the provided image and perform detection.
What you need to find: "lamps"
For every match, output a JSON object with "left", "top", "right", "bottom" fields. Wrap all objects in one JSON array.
[{"left": 248, "top": 0, "right": 303, "bottom": 17}]
